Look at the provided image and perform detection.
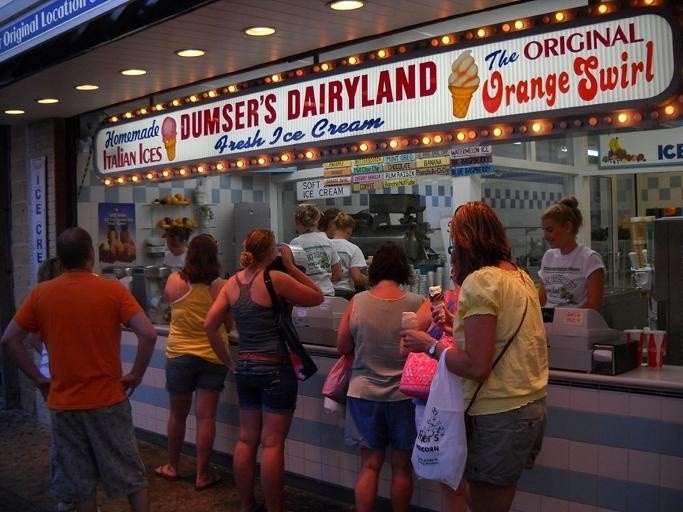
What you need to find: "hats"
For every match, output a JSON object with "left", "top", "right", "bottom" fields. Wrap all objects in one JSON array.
[{"left": 161, "top": 227, "right": 189, "bottom": 241}]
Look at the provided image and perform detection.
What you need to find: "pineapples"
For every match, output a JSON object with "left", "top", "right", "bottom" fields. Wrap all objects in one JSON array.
[{"left": 107, "top": 216, "right": 130, "bottom": 242}]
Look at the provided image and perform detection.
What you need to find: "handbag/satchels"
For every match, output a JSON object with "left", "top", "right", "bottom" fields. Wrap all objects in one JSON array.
[
  {"left": 400, "top": 336, "right": 456, "bottom": 399},
  {"left": 278, "top": 315, "right": 317, "bottom": 381},
  {"left": 323, "top": 352, "right": 353, "bottom": 403}
]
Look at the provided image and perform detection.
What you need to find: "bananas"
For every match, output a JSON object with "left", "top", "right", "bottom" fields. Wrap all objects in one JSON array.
[{"left": 609, "top": 136, "right": 620, "bottom": 152}]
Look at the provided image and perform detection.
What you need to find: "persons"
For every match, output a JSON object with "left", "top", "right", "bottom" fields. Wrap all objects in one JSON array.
[
  {"left": 162, "top": 224, "right": 193, "bottom": 271},
  {"left": 204, "top": 226, "right": 325, "bottom": 510},
  {"left": 2, "top": 225, "right": 158, "bottom": 510},
  {"left": 287, "top": 202, "right": 369, "bottom": 300},
  {"left": 152, "top": 234, "right": 233, "bottom": 490},
  {"left": 335, "top": 197, "right": 551, "bottom": 512},
  {"left": 535, "top": 195, "right": 606, "bottom": 313}
]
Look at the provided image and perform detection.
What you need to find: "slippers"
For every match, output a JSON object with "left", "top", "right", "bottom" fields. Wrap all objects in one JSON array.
[
  {"left": 194, "top": 470, "right": 224, "bottom": 491},
  {"left": 154, "top": 465, "right": 180, "bottom": 481}
]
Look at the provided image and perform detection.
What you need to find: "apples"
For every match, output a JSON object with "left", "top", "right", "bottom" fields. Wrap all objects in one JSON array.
[
  {"left": 164, "top": 193, "right": 191, "bottom": 203},
  {"left": 625, "top": 154, "right": 644, "bottom": 160},
  {"left": 158, "top": 217, "right": 197, "bottom": 228}
]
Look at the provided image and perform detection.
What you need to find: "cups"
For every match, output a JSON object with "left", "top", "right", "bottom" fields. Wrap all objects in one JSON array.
[
  {"left": 403, "top": 263, "right": 444, "bottom": 302},
  {"left": 623, "top": 330, "right": 668, "bottom": 367}
]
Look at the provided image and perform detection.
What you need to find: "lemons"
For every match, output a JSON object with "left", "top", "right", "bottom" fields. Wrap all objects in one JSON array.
[{"left": 115, "top": 241, "right": 124, "bottom": 254}]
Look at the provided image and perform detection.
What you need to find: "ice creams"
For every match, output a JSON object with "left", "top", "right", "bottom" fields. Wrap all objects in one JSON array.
[
  {"left": 428, "top": 286, "right": 446, "bottom": 311},
  {"left": 449, "top": 50, "right": 481, "bottom": 119},
  {"left": 161, "top": 116, "right": 178, "bottom": 161},
  {"left": 398, "top": 311, "right": 420, "bottom": 333}
]
